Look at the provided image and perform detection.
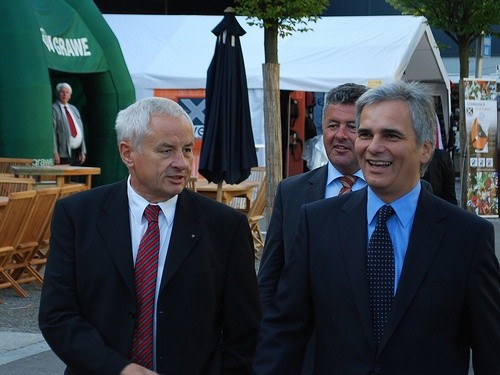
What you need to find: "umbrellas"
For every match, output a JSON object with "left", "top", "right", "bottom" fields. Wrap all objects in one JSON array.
[{"left": 198, "top": 7, "right": 259, "bottom": 202}]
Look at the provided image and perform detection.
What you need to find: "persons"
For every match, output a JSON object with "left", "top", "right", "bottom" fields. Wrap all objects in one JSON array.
[
  {"left": 422, "top": 147, "right": 458, "bottom": 205},
  {"left": 258, "top": 81, "right": 433, "bottom": 375},
  {"left": 38, "top": 96, "right": 258, "bottom": 375},
  {"left": 252, "top": 82, "right": 500, "bottom": 375},
  {"left": 52, "top": 83, "right": 87, "bottom": 165}
]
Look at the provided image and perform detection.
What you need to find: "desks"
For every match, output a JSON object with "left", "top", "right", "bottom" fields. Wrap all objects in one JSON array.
[
  {"left": 195, "top": 180, "right": 259, "bottom": 213},
  {"left": 10, "top": 164, "right": 101, "bottom": 189}
]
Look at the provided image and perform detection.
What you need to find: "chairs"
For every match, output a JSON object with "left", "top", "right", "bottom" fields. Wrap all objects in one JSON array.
[
  {"left": 0, "top": 158, "right": 91, "bottom": 304},
  {"left": 245, "top": 174, "right": 266, "bottom": 260},
  {"left": 184, "top": 178, "right": 197, "bottom": 193}
]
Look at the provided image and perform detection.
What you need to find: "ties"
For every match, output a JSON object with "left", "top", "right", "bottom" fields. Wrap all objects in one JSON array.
[
  {"left": 64, "top": 106, "right": 77, "bottom": 137},
  {"left": 367, "top": 205, "right": 396, "bottom": 354},
  {"left": 338, "top": 175, "right": 360, "bottom": 195},
  {"left": 134, "top": 204, "right": 162, "bottom": 371}
]
